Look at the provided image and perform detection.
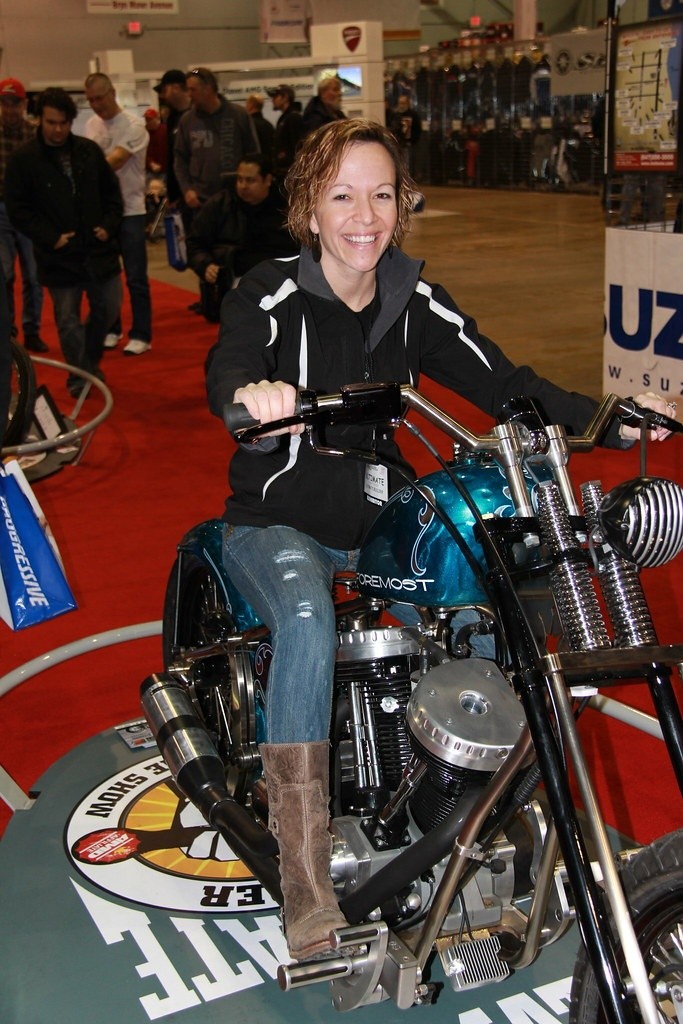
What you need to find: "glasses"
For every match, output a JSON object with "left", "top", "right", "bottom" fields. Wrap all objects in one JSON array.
[{"left": 87, "top": 90, "right": 113, "bottom": 103}]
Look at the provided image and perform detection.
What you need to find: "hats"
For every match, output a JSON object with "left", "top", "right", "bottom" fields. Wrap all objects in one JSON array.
[
  {"left": 263, "top": 84, "right": 295, "bottom": 98},
  {"left": 142, "top": 109, "right": 158, "bottom": 119},
  {"left": 1, "top": 77, "right": 28, "bottom": 101},
  {"left": 152, "top": 69, "right": 186, "bottom": 92}
]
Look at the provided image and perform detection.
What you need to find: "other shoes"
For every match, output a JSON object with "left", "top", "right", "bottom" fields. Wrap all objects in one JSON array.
[{"left": 67, "top": 374, "right": 81, "bottom": 387}]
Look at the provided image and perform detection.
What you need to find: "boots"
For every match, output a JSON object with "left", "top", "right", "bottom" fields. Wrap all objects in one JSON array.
[{"left": 256, "top": 741, "right": 351, "bottom": 960}]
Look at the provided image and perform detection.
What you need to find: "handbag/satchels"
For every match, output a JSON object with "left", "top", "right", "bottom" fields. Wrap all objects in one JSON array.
[{"left": 164, "top": 210, "right": 188, "bottom": 272}]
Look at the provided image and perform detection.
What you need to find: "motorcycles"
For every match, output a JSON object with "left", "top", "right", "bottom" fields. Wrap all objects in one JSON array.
[{"left": 133, "top": 376, "right": 682, "bottom": 1024}]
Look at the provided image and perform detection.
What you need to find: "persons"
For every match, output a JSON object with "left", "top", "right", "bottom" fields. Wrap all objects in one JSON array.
[
  {"left": 0, "top": 67, "right": 347, "bottom": 400},
  {"left": 384, "top": 95, "right": 422, "bottom": 176},
  {"left": 201, "top": 118, "right": 677, "bottom": 961}
]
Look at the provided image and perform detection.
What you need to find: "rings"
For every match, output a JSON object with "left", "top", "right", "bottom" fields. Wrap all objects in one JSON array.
[{"left": 667, "top": 401, "right": 677, "bottom": 409}]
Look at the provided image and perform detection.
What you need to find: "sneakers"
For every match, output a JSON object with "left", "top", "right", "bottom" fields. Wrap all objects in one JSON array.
[
  {"left": 23, "top": 334, "right": 49, "bottom": 352},
  {"left": 95, "top": 368, "right": 105, "bottom": 383},
  {"left": 123, "top": 338, "right": 152, "bottom": 354},
  {"left": 103, "top": 331, "right": 124, "bottom": 349}
]
